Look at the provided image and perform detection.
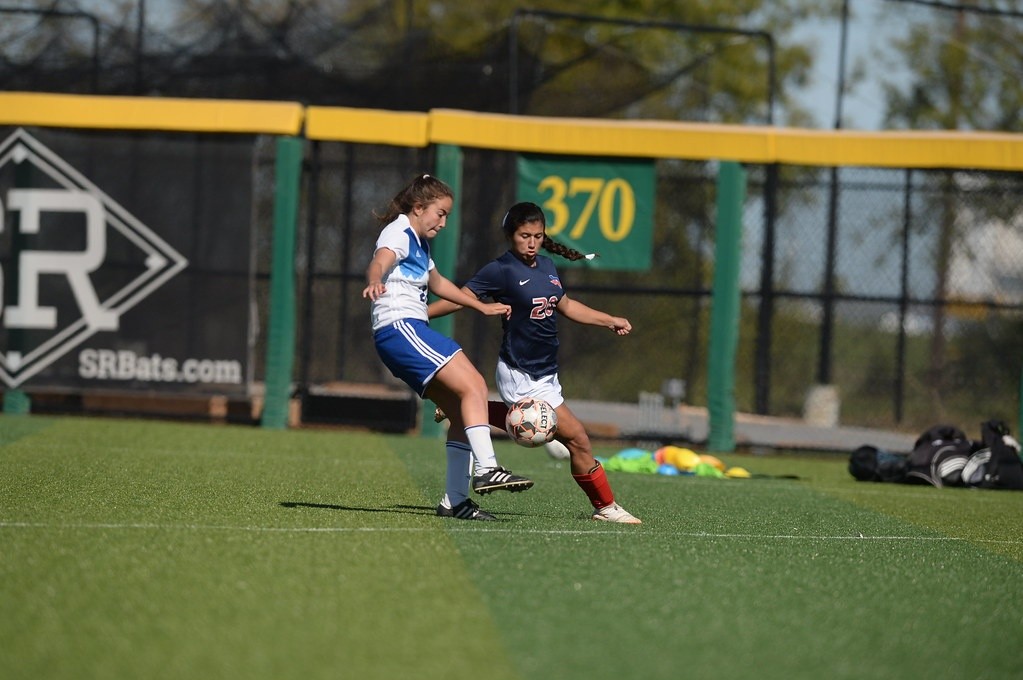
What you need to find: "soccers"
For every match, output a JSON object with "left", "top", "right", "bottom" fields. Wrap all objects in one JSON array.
[{"left": 505, "top": 396, "right": 558, "bottom": 448}]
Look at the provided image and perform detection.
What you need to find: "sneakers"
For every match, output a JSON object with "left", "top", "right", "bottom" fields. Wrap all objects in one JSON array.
[
  {"left": 437, "top": 498, "right": 498, "bottom": 521},
  {"left": 591, "top": 501, "right": 642, "bottom": 524},
  {"left": 472, "top": 466, "right": 534, "bottom": 496},
  {"left": 434, "top": 406, "right": 447, "bottom": 423}
]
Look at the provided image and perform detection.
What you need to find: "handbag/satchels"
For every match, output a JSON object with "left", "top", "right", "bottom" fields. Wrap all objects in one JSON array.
[{"left": 849, "top": 419, "right": 1023, "bottom": 491}]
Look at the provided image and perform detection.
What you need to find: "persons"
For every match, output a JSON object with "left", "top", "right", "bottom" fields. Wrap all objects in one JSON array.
[
  {"left": 427, "top": 202, "right": 642, "bottom": 524},
  {"left": 362, "top": 173, "right": 535, "bottom": 521}
]
